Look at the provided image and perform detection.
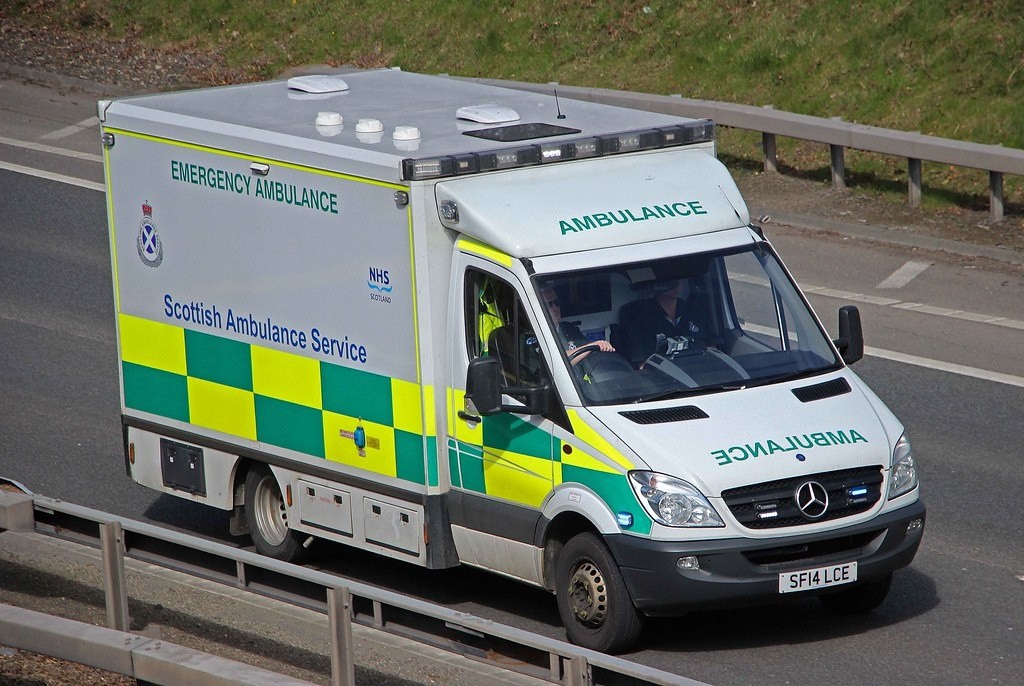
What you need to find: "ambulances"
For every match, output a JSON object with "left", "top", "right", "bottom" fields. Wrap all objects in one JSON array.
[{"left": 98, "top": 66, "right": 929, "bottom": 657}]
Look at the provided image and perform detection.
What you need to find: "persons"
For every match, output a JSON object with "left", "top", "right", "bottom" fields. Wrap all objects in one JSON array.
[
  {"left": 514, "top": 282, "right": 616, "bottom": 390},
  {"left": 632, "top": 270, "right": 727, "bottom": 370}
]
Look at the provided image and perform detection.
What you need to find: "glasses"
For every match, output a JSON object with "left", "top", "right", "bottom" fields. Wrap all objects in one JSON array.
[{"left": 548, "top": 299, "right": 562, "bottom": 307}]
